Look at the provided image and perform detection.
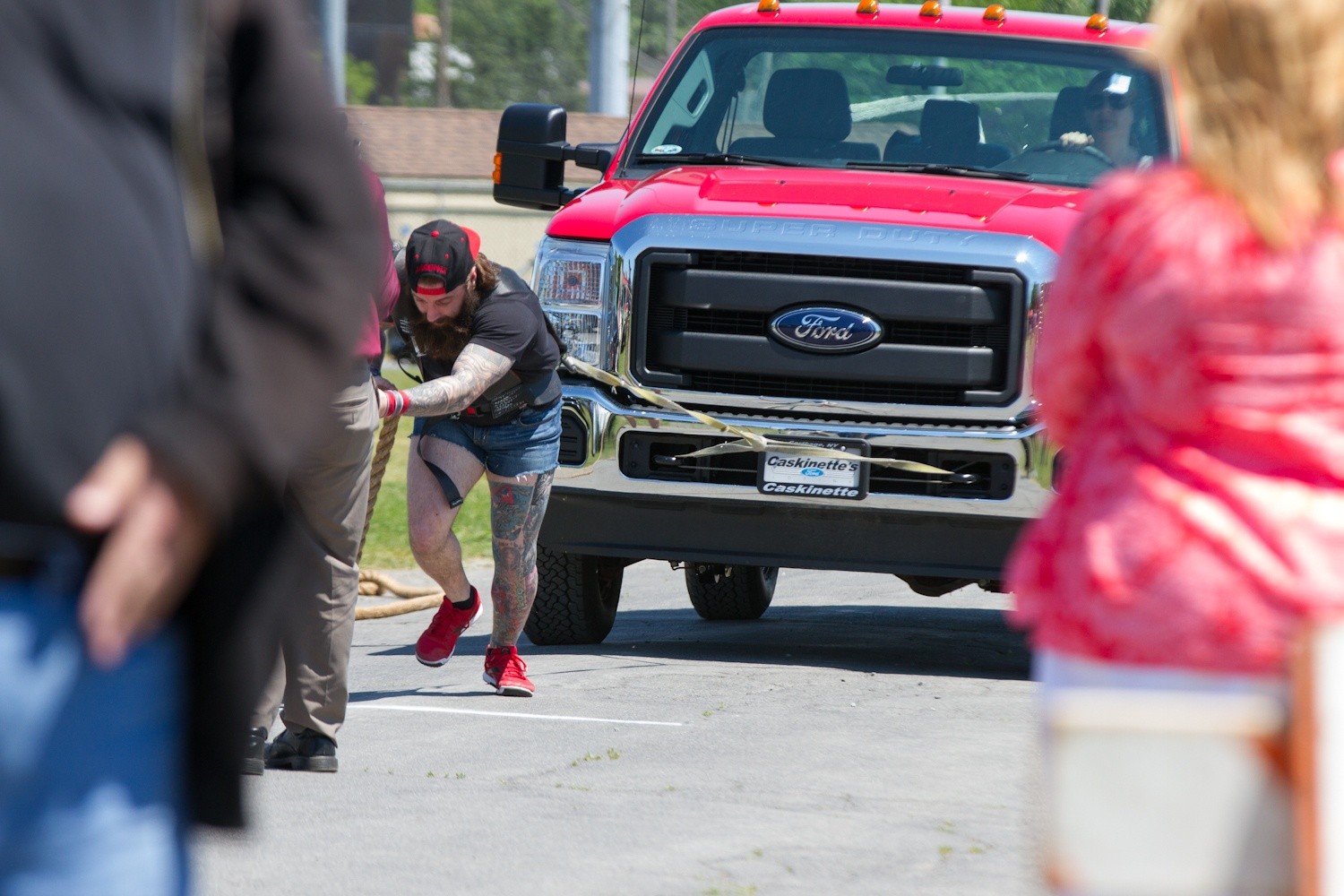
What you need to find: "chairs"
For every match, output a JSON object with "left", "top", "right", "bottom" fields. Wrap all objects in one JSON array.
[{"left": 731, "top": 66, "right": 1093, "bottom": 169}]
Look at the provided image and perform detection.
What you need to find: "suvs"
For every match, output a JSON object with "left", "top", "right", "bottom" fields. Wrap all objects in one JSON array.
[{"left": 487, "top": 1, "right": 1189, "bottom": 654}]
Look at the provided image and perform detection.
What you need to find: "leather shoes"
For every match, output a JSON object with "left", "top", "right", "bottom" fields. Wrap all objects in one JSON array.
[
  {"left": 266, "top": 730, "right": 339, "bottom": 770},
  {"left": 240, "top": 726, "right": 269, "bottom": 775}
]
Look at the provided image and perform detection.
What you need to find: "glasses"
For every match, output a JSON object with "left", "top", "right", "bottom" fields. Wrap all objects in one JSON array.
[{"left": 1085, "top": 93, "right": 1134, "bottom": 110}]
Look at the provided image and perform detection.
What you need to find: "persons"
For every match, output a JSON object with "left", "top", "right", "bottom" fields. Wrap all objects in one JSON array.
[
  {"left": 375, "top": 219, "right": 567, "bottom": 696},
  {"left": 0, "top": 1, "right": 401, "bottom": 896},
  {"left": 1057, "top": 69, "right": 1157, "bottom": 176},
  {"left": 1007, "top": 0, "right": 1344, "bottom": 895}
]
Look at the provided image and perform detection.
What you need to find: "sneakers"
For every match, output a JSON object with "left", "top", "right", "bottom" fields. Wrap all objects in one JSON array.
[
  {"left": 416, "top": 586, "right": 483, "bottom": 668},
  {"left": 483, "top": 645, "right": 535, "bottom": 698}
]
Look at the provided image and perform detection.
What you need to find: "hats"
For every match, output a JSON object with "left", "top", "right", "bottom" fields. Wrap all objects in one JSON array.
[{"left": 405, "top": 220, "right": 480, "bottom": 294}]
